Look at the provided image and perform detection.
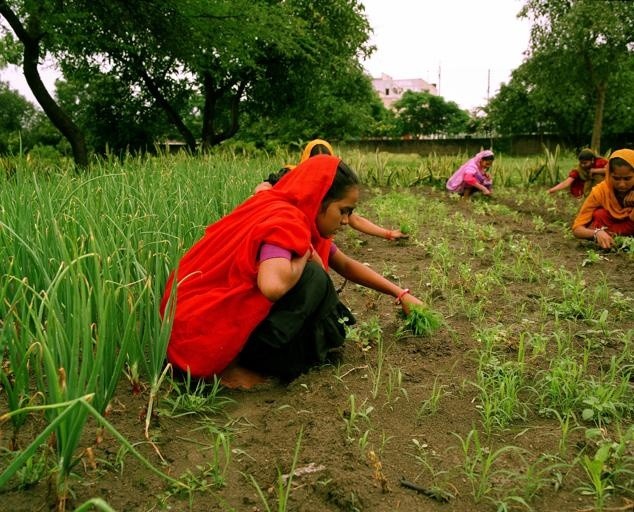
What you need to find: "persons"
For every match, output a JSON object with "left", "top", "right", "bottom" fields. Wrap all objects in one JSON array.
[
  {"left": 446, "top": 149, "right": 495, "bottom": 200},
  {"left": 546, "top": 149, "right": 608, "bottom": 197},
  {"left": 161, "top": 152, "right": 428, "bottom": 392},
  {"left": 572, "top": 149, "right": 634, "bottom": 250},
  {"left": 255, "top": 138, "right": 409, "bottom": 242}
]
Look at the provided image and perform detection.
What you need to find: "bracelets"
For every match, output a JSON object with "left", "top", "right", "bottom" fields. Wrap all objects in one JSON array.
[
  {"left": 594, "top": 228, "right": 601, "bottom": 237},
  {"left": 395, "top": 289, "right": 410, "bottom": 305},
  {"left": 385, "top": 230, "right": 392, "bottom": 240},
  {"left": 308, "top": 244, "right": 313, "bottom": 264},
  {"left": 480, "top": 185, "right": 484, "bottom": 190}
]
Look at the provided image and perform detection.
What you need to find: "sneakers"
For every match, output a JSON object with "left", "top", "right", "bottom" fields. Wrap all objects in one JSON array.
[{"left": 210, "top": 366, "right": 273, "bottom": 393}]
